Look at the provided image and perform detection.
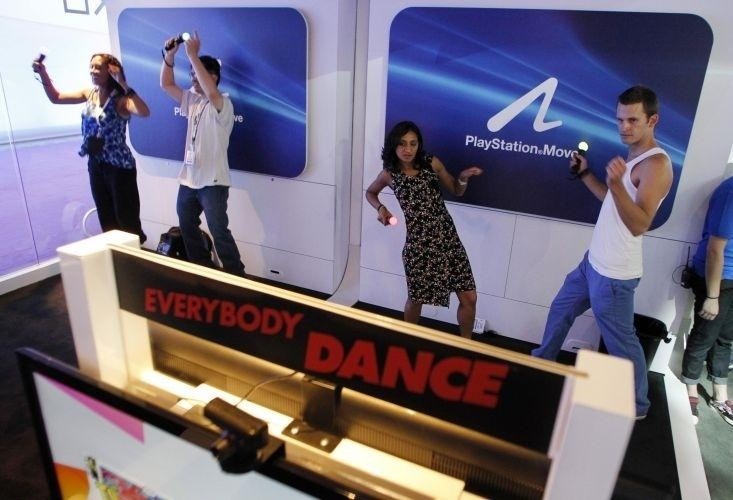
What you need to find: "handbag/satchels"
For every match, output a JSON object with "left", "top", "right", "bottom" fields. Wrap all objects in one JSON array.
[{"left": 156, "top": 226, "right": 212, "bottom": 265}]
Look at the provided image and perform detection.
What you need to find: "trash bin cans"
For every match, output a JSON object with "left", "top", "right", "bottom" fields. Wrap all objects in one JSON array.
[{"left": 599, "top": 313, "right": 671, "bottom": 373}]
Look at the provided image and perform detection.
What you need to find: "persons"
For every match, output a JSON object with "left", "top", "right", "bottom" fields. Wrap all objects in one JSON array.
[
  {"left": 528, "top": 87, "right": 674, "bottom": 419},
  {"left": 681, "top": 176, "right": 732, "bottom": 426},
  {"left": 35, "top": 51, "right": 147, "bottom": 244},
  {"left": 366, "top": 121, "right": 483, "bottom": 338},
  {"left": 159, "top": 29, "right": 246, "bottom": 275}
]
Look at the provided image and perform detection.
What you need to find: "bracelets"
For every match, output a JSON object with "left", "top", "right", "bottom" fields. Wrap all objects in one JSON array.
[
  {"left": 456, "top": 179, "right": 468, "bottom": 185},
  {"left": 707, "top": 296, "right": 720, "bottom": 300},
  {"left": 124, "top": 87, "right": 135, "bottom": 97},
  {"left": 376, "top": 204, "right": 383, "bottom": 210},
  {"left": 576, "top": 169, "right": 589, "bottom": 180}
]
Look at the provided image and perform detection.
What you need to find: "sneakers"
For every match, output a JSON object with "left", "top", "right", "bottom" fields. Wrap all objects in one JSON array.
[
  {"left": 708, "top": 395, "right": 733, "bottom": 426},
  {"left": 689, "top": 395, "right": 699, "bottom": 425}
]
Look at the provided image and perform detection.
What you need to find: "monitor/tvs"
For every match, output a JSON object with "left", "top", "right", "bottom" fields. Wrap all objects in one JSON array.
[{"left": 14, "top": 346, "right": 395, "bottom": 500}]
